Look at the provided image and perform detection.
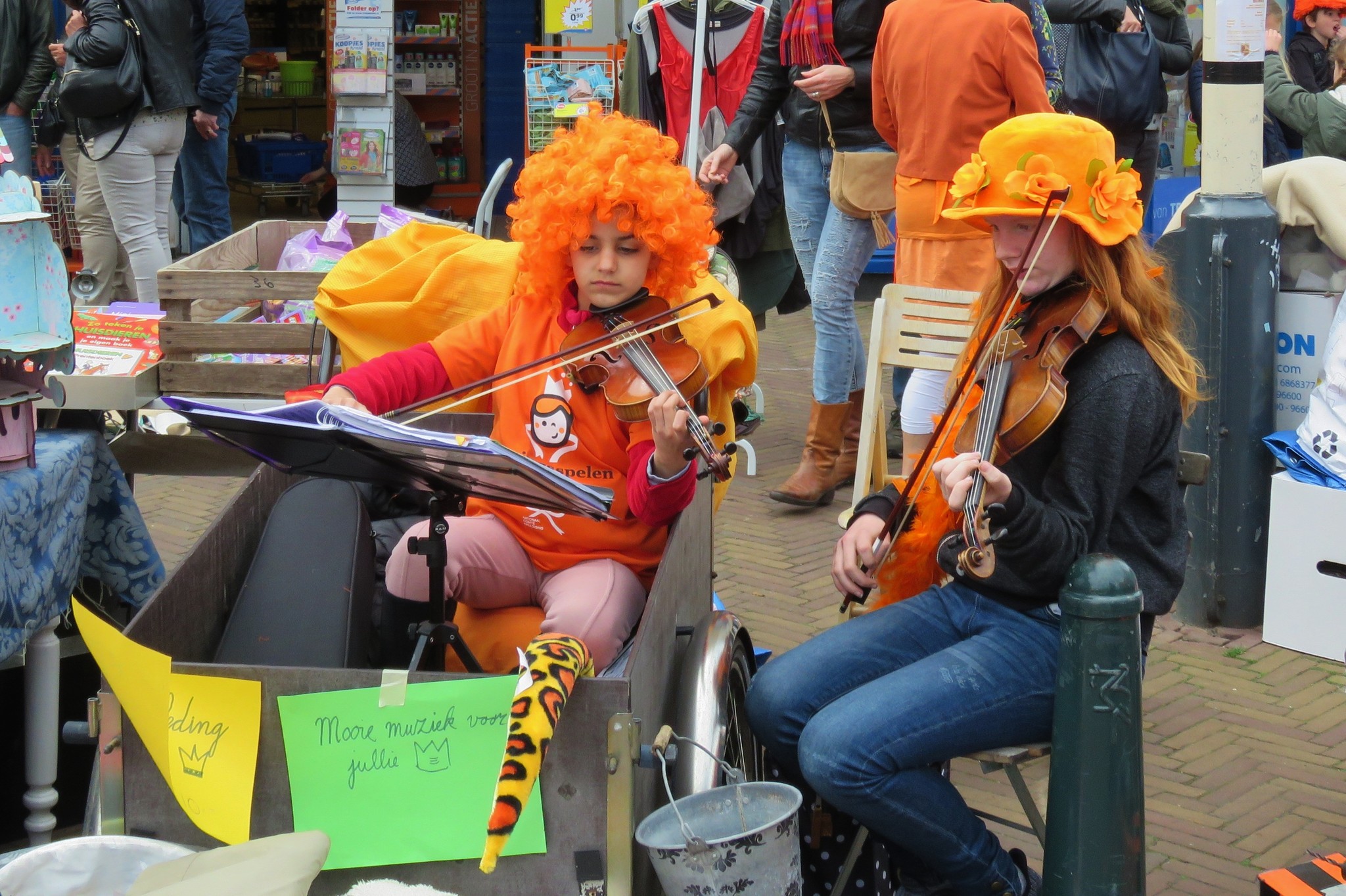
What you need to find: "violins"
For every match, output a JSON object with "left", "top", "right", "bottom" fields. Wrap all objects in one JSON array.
[
  {"left": 559, "top": 295, "right": 737, "bottom": 482},
  {"left": 946, "top": 281, "right": 1108, "bottom": 582}
]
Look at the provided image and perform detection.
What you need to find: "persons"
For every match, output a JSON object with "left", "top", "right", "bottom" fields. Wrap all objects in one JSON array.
[
  {"left": 1286, "top": 0, "right": 1346, "bottom": 94},
  {"left": 698, "top": 0, "right": 899, "bottom": 507},
  {"left": 317, "top": 112, "right": 722, "bottom": 676},
  {"left": 1263, "top": 28, "right": 1346, "bottom": 162},
  {"left": 869, "top": 0, "right": 1064, "bottom": 479},
  {"left": 742, "top": 112, "right": 1213, "bottom": 896},
  {"left": 1043, "top": 0, "right": 1194, "bottom": 228},
  {"left": 172, "top": 0, "right": 252, "bottom": 255},
  {"left": 35, "top": 0, "right": 203, "bottom": 307},
  {"left": 1187, "top": 0, "right": 1290, "bottom": 169},
  {"left": 298, "top": 88, "right": 440, "bottom": 222},
  {"left": 0, "top": 0, "right": 57, "bottom": 180}
]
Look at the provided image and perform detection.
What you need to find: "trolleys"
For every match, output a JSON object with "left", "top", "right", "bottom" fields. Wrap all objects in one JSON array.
[
  {"left": 224, "top": 76, "right": 316, "bottom": 219},
  {"left": 156, "top": 156, "right": 514, "bottom": 402}
]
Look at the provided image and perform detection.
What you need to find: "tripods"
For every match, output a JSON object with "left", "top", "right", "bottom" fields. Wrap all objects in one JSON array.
[{"left": 169, "top": 422, "right": 612, "bottom": 673}]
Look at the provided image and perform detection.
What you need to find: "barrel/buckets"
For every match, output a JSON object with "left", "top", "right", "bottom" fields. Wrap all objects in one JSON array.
[
  {"left": 279, "top": 61, "right": 317, "bottom": 96},
  {"left": 636, "top": 726, "right": 804, "bottom": 896}
]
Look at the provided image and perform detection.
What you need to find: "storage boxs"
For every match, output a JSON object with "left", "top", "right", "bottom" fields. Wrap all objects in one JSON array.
[
  {"left": 1273, "top": 290, "right": 1346, "bottom": 469},
  {"left": 231, "top": 134, "right": 329, "bottom": 184},
  {"left": 267, "top": 69, "right": 281, "bottom": 82},
  {"left": 157, "top": 219, "right": 474, "bottom": 394},
  {"left": 32, "top": 359, "right": 162, "bottom": 410},
  {"left": 247, "top": 47, "right": 287, "bottom": 62},
  {"left": 415, "top": 24, "right": 440, "bottom": 36},
  {"left": 1262, "top": 471, "right": 1345, "bottom": 662}
]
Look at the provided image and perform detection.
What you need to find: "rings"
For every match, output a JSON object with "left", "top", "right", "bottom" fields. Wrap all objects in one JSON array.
[
  {"left": 205, "top": 129, "right": 213, "bottom": 134},
  {"left": 812, "top": 92, "right": 820, "bottom": 98},
  {"left": 1126, "top": 29, "right": 1132, "bottom": 33}
]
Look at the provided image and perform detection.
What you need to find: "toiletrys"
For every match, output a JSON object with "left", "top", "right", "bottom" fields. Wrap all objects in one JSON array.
[
  {"left": 448, "top": 13, "right": 458, "bottom": 37},
  {"left": 395, "top": 12, "right": 403, "bottom": 36},
  {"left": 439, "top": 12, "right": 448, "bottom": 36},
  {"left": 404, "top": 10, "right": 417, "bottom": 36},
  {"left": 394, "top": 52, "right": 458, "bottom": 87}
]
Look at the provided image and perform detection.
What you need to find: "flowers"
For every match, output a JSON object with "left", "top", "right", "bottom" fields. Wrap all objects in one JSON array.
[
  {"left": 947, "top": 152, "right": 991, "bottom": 210},
  {"left": 1085, "top": 158, "right": 1143, "bottom": 224},
  {"left": 1004, "top": 152, "right": 1071, "bottom": 207}
]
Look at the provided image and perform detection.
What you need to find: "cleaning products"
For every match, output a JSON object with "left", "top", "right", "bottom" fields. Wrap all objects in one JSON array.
[
  {"left": 447, "top": 143, "right": 467, "bottom": 182},
  {"left": 435, "top": 147, "right": 448, "bottom": 181}
]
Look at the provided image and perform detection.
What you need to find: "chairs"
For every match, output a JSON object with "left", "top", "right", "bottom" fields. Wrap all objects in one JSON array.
[
  {"left": 826, "top": 451, "right": 1210, "bottom": 896},
  {"left": 838, "top": 283, "right": 983, "bottom": 627}
]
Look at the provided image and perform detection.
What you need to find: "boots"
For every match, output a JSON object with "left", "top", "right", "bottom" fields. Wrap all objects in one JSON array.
[
  {"left": 827, "top": 389, "right": 874, "bottom": 489},
  {"left": 768, "top": 395, "right": 855, "bottom": 508}
]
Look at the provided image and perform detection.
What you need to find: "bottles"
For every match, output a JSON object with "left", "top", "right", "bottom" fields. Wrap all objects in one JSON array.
[
  {"left": 425, "top": 54, "right": 435, "bottom": 85},
  {"left": 433, "top": 147, "right": 466, "bottom": 184},
  {"left": 435, "top": 53, "right": 445, "bottom": 85},
  {"left": 404, "top": 53, "right": 414, "bottom": 73},
  {"left": 414, "top": 53, "right": 425, "bottom": 74},
  {"left": 445, "top": 54, "right": 456, "bottom": 85},
  {"left": 394, "top": 53, "right": 404, "bottom": 73}
]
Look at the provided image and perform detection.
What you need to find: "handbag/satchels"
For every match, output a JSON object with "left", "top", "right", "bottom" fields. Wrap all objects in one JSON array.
[
  {"left": 59, "top": 19, "right": 141, "bottom": 103},
  {"left": 37, "top": 100, "right": 66, "bottom": 147},
  {"left": 829, "top": 150, "right": 899, "bottom": 247},
  {"left": 680, "top": 107, "right": 754, "bottom": 230}
]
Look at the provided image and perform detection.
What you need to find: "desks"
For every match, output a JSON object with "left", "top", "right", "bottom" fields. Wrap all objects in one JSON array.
[{"left": 0, "top": 428, "right": 164, "bottom": 846}]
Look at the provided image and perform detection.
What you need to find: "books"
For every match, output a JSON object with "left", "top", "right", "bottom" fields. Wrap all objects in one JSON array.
[
  {"left": 158, "top": 394, "right": 618, "bottom": 521},
  {"left": 337, "top": 126, "right": 385, "bottom": 175},
  {"left": 23, "top": 311, "right": 165, "bottom": 377},
  {"left": 333, "top": 31, "right": 387, "bottom": 95}
]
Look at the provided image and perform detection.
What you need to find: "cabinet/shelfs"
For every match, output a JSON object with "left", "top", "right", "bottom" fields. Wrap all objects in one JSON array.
[
  {"left": 394, "top": 0, "right": 463, "bottom": 157},
  {"left": 0, "top": 169, "right": 75, "bottom": 408}
]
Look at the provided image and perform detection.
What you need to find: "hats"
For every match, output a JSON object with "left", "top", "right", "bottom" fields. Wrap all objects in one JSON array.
[
  {"left": 939, "top": 113, "right": 1145, "bottom": 246},
  {"left": 1292, "top": 0, "right": 1346, "bottom": 21}
]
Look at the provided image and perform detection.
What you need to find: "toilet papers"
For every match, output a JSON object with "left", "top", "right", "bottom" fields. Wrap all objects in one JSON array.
[{"left": 268, "top": 72, "right": 281, "bottom": 82}]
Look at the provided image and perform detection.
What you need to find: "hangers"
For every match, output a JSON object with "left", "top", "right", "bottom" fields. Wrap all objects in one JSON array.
[{"left": 632, "top": 0, "right": 770, "bottom": 35}]
